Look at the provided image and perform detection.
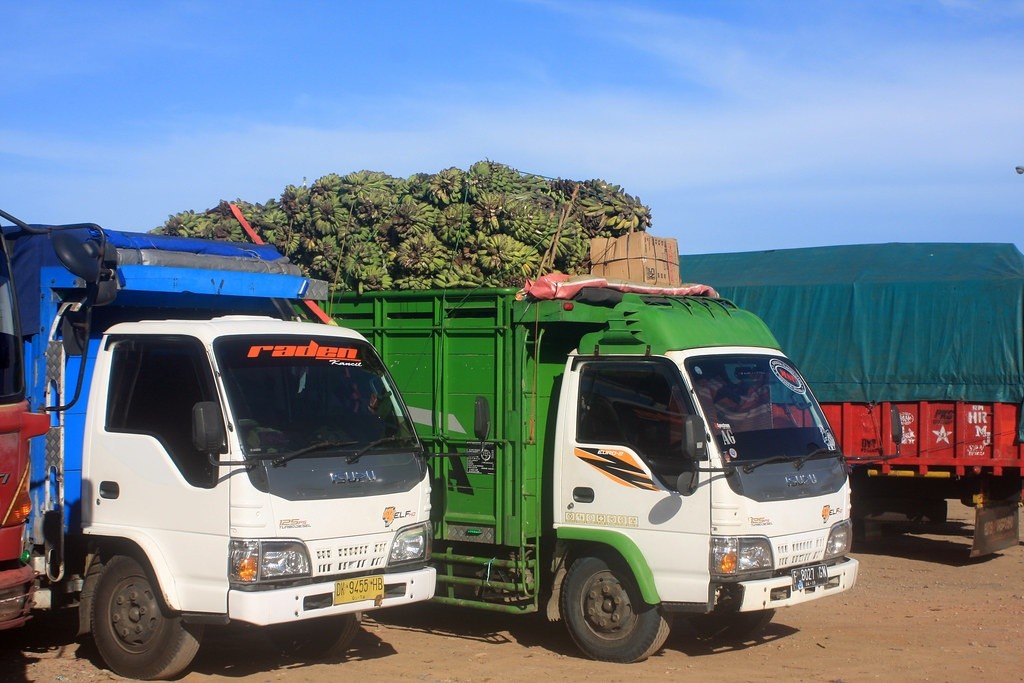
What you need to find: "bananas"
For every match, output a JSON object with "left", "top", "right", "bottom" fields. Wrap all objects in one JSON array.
[{"left": 144, "top": 159, "right": 652, "bottom": 291}]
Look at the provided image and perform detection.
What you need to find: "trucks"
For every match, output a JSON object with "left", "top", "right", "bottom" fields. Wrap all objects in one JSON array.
[
  {"left": 0, "top": 208, "right": 107, "bottom": 631},
  {"left": 679, "top": 242, "right": 1023, "bottom": 558},
  {"left": 289, "top": 277, "right": 904, "bottom": 664},
  {"left": 3, "top": 222, "right": 491, "bottom": 681}
]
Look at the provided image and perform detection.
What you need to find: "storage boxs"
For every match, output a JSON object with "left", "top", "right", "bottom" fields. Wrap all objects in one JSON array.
[{"left": 590, "top": 232, "right": 680, "bottom": 286}]
